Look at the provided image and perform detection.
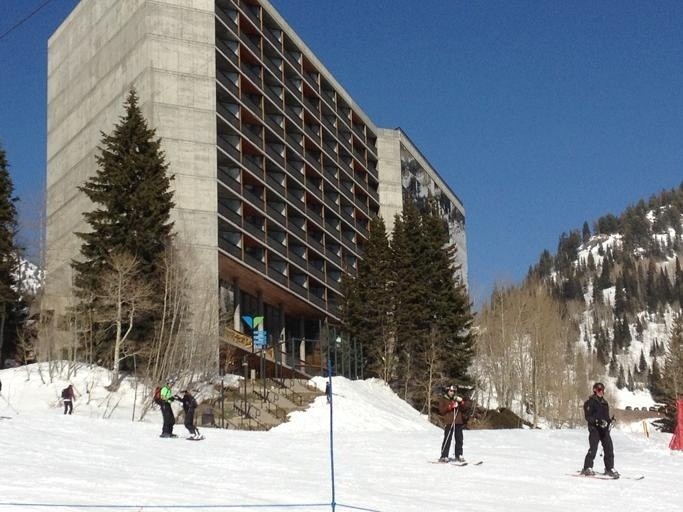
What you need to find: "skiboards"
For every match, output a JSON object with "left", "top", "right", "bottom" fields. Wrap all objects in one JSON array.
[
  {"left": 428, "top": 461, "right": 483, "bottom": 466},
  {"left": 565, "top": 471, "right": 644, "bottom": 480}
]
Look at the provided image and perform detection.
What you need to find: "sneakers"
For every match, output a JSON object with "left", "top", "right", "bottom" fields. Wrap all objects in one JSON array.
[
  {"left": 458, "top": 455, "right": 464, "bottom": 461},
  {"left": 187, "top": 434, "right": 202, "bottom": 439},
  {"left": 583, "top": 467, "right": 595, "bottom": 476},
  {"left": 604, "top": 467, "right": 619, "bottom": 478},
  {"left": 440, "top": 457, "right": 449, "bottom": 463}
]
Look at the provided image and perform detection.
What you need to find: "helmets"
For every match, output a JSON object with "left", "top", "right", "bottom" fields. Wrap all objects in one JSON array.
[
  {"left": 180, "top": 387, "right": 187, "bottom": 392},
  {"left": 446, "top": 384, "right": 457, "bottom": 392},
  {"left": 167, "top": 379, "right": 175, "bottom": 385},
  {"left": 592, "top": 382, "right": 605, "bottom": 392}
]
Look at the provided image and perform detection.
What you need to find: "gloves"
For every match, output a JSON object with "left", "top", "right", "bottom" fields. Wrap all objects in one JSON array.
[
  {"left": 456, "top": 397, "right": 463, "bottom": 402},
  {"left": 449, "top": 402, "right": 458, "bottom": 409},
  {"left": 596, "top": 419, "right": 609, "bottom": 428}
]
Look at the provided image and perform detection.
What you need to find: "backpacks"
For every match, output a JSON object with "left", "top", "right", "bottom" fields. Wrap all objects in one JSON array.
[{"left": 153, "top": 386, "right": 165, "bottom": 406}]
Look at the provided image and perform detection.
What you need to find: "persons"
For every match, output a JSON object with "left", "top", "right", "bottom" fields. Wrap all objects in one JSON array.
[
  {"left": 438, "top": 383, "right": 470, "bottom": 463},
  {"left": 324, "top": 381, "right": 330, "bottom": 404},
  {"left": 175, "top": 387, "right": 204, "bottom": 441},
  {"left": 159, "top": 377, "right": 178, "bottom": 438},
  {"left": 61, "top": 384, "right": 76, "bottom": 415},
  {"left": 581, "top": 382, "right": 619, "bottom": 479}
]
